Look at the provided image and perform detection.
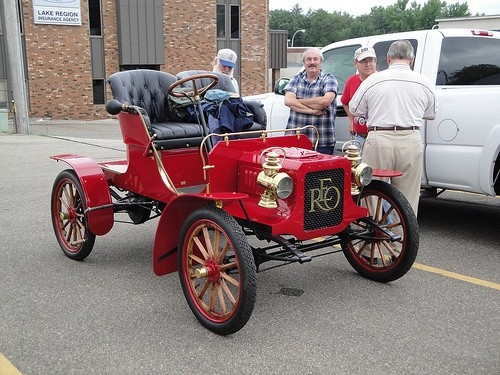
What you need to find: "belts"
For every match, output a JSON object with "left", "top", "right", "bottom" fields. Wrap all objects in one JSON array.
[
  {"left": 357, "top": 134, "right": 367, "bottom": 137},
  {"left": 368, "top": 126, "right": 419, "bottom": 131}
]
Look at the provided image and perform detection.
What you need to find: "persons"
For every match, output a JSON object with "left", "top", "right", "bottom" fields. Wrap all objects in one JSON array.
[
  {"left": 348, "top": 39, "right": 439, "bottom": 265},
  {"left": 283, "top": 47, "right": 339, "bottom": 156},
  {"left": 210, "top": 48, "right": 239, "bottom": 96},
  {"left": 341, "top": 46, "right": 396, "bottom": 231}
]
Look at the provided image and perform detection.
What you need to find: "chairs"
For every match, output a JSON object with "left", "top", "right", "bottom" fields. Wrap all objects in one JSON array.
[{"left": 106, "top": 69, "right": 267, "bottom": 151}]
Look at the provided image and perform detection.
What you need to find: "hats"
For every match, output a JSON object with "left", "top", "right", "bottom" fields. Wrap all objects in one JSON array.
[
  {"left": 218, "top": 48, "right": 237, "bottom": 68},
  {"left": 354, "top": 47, "right": 376, "bottom": 61}
]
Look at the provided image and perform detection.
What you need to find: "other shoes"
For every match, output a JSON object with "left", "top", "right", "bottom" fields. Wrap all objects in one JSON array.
[
  {"left": 362, "top": 254, "right": 379, "bottom": 264},
  {"left": 392, "top": 256, "right": 397, "bottom": 262}
]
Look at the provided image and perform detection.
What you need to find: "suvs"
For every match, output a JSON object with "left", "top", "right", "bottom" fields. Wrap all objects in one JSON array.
[{"left": 240, "top": 28, "right": 500, "bottom": 204}]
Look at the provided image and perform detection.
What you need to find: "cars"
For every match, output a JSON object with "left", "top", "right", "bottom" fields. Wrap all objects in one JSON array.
[{"left": 47, "top": 66, "right": 421, "bottom": 339}]
